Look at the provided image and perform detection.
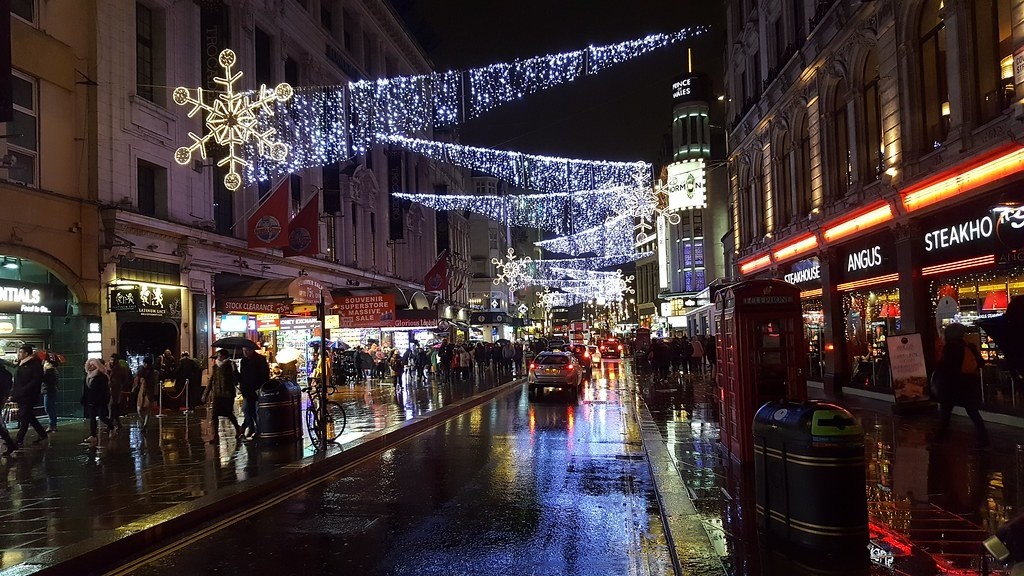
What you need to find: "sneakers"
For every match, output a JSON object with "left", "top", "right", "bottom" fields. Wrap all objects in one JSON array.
[
  {"left": 84, "top": 435, "right": 97, "bottom": 442},
  {"left": 110, "top": 426, "right": 116, "bottom": 438}
]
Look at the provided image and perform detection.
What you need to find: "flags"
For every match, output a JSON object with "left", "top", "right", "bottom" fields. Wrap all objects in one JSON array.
[
  {"left": 246, "top": 177, "right": 319, "bottom": 257},
  {"left": 424, "top": 253, "right": 447, "bottom": 291}
]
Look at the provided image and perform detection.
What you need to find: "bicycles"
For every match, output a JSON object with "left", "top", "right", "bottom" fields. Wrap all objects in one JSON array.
[{"left": 301, "top": 383, "right": 346, "bottom": 450}]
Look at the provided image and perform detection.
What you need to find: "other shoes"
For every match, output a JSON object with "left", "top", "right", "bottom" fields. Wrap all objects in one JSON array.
[
  {"left": 33, "top": 435, "right": 48, "bottom": 442},
  {"left": 210, "top": 435, "right": 219, "bottom": 445},
  {"left": 3, "top": 445, "right": 18, "bottom": 456},
  {"left": 235, "top": 427, "right": 244, "bottom": 438},
  {"left": 247, "top": 429, "right": 256, "bottom": 440},
  {"left": 45, "top": 426, "right": 56, "bottom": 432}
]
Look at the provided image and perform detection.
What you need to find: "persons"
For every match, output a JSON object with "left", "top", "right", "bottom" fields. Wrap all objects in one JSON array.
[
  {"left": 0, "top": 347, "right": 268, "bottom": 453},
  {"left": 278, "top": 360, "right": 297, "bottom": 381},
  {"left": 312, "top": 340, "right": 525, "bottom": 387},
  {"left": 934, "top": 323, "right": 986, "bottom": 436},
  {"left": 644, "top": 334, "right": 718, "bottom": 380}
]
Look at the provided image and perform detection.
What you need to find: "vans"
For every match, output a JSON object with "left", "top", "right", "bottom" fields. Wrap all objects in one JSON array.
[{"left": 548, "top": 340, "right": 565, "bottom": 350}]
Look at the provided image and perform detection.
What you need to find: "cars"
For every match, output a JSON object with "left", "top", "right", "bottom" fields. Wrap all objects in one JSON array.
[
  {"left": 587, "top": 346, "right": 601, "bottom": 366},
  {"left": 528, "top": 351, "right": 582, "bottom": 392},
  {"left": 599, "top": 342, "right": 622, "bottom": 358}
]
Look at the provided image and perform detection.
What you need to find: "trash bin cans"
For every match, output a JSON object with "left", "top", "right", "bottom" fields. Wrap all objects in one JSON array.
[
  {"left": 751, "top": 399, "right": 872, "bottom": 567},
  {"left": 259, "top": 379, "right": 305, "bottom": 463}
]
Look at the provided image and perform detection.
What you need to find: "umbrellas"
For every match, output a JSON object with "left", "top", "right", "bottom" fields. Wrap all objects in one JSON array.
[
  {"left": 360, "top": 353, "right": 374, "bottom": 368},
  {"left": 327, "top": 339, "right": 349, "bottom": 349},
  {"left": 34, "top": 349, "right": 64, "bottom": 361},
  {"left": 494, "top": 339, "right": 510, "bottom": 344},
  {"left": 210, "top": 337, "right": 258, "bottom": 364},
  {"left": 210, "top": 350, "right": 243, "bottom": 359},
  {"left": 277, "top": 347, "right": 300, "bottom": 363},
  {"left": 308, "top": 337, "right": 334, "bottom": 347}
]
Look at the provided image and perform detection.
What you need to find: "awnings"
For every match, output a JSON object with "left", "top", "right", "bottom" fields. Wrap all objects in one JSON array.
[
  {"left": 219, "top": 275, "right": 334, "bottom": 306},
  {"left": 449, "top": 321, "right": 481, "bottom": 332}
]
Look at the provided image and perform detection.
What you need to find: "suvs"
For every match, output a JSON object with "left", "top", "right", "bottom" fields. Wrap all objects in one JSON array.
[{"left": 561, "top": 344, "right": 593, "bottom": 375}]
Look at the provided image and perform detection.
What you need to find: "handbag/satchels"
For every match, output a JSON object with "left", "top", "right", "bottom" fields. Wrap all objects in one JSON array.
[{"left": 393, "top": 363, "right": 401, "bottom": 371}]
